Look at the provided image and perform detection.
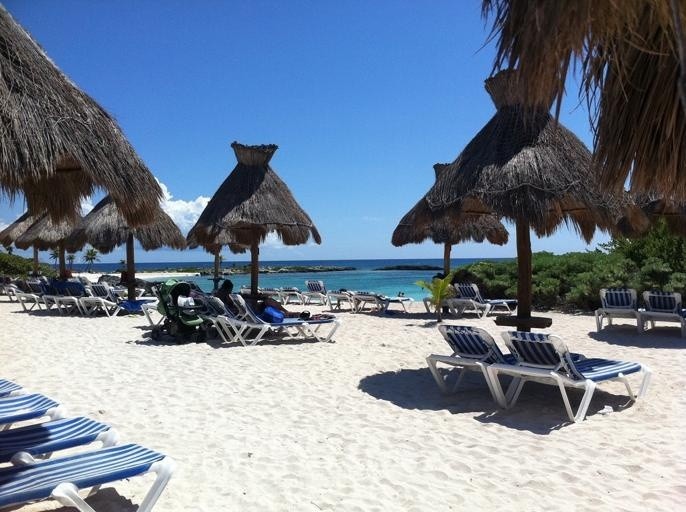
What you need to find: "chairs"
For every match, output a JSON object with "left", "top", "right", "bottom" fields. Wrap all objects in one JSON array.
[
  {"left": 0, "top": 375, "right": 173, "bottom": 511},
  {"left": 5, "top": 279, "right": 158, "bottom": 318},
  {"left": 262, "top": 280, "right": 326, "bottom": 306},
  {"left": 327, "top": 290, "right": 411, "bottom": 316},
  {"left": 190, "top": 290, "right": 339, "bottom": 346},
  {"left": 136, "top": 296, "right": 168, "bottom": 328},
  {"left": 425, "top": 281, "right": 686, "bottom": 424}
]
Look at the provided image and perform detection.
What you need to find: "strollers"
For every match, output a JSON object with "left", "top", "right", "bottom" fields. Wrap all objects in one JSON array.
[{"left": 151, "top": 278, "right": 204, "bottom": 342}]
